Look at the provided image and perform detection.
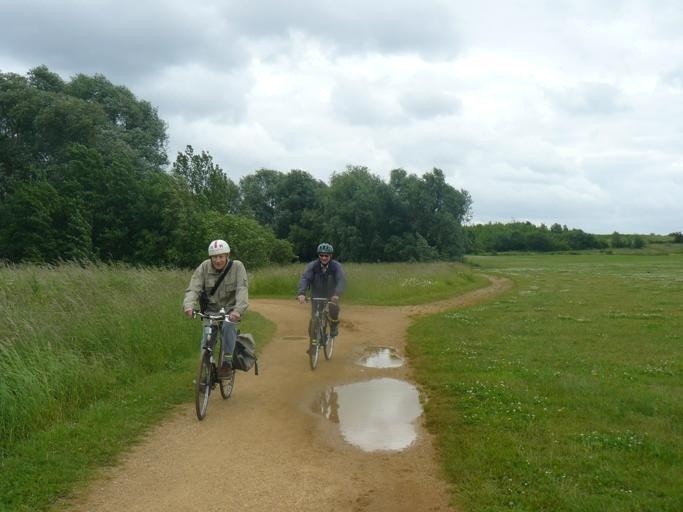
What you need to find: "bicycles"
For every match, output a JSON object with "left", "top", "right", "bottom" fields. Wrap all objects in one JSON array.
[
  {"left": 295, "top": 295, "right": 334, "bottom": 370},
  {"left": 184, "top": 309, "right": 237, "bottom": 424}
]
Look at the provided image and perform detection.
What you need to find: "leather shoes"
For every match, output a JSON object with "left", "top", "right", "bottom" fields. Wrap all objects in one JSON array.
[{"left": 216, "top": 361, "right": 233, "bottom": 380}]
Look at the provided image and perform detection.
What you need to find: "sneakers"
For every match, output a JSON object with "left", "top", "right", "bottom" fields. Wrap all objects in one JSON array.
[{"left": 329, "top": 324, "right": 338, "bottom": 336}]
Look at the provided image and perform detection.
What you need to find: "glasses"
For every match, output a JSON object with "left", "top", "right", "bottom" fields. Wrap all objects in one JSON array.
[{"left": 320, "top": 254, "right": 329, "bottom": 257}]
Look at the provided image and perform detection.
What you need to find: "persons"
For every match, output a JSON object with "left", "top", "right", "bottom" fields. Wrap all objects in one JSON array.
[
  {"left": 298, "top": 243, "right": 343, "bottom": 354},
  {"left": 183, "top": 239, "right": 249, "bottom": 386}
]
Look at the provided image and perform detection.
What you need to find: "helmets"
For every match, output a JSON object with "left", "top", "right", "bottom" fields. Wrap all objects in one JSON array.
[
  {"left": 208, "top": 240, "right": 231, "bottom": 257},
  {"left": 317, "top": 243, "right": 333, "bottom": 254}
]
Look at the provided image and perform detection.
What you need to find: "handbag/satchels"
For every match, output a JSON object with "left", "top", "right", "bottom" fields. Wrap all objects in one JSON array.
[
  {"left": 233, "top": 334, "right": 258, "bottom": 376},
  {"left": 199, "top": 293, "right": 209, "bottom": 321}
]
